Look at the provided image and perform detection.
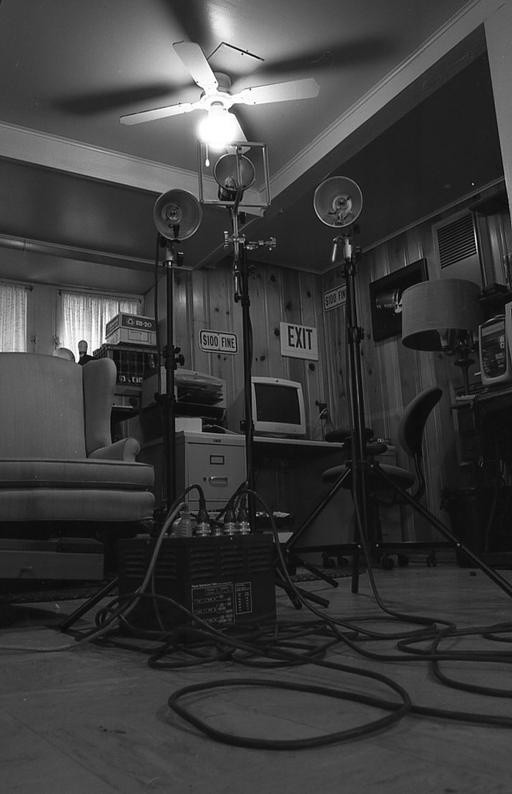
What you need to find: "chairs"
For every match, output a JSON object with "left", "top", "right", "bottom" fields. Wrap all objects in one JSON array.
[
  {"left": 319, "top": 387, "right": 444, "bottom": 569},
  {"left": 0, "top": 350, "right": 155, "bottom": 585}
]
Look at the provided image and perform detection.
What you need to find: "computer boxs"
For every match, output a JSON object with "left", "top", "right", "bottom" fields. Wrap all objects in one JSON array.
[{"left": 478, "top": 314, "right": 512, "bottom": 386}]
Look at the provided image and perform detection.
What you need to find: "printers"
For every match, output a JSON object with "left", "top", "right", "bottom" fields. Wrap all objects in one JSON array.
[{"left": 141, "top": 364, "right": 227, "bottom": 409}]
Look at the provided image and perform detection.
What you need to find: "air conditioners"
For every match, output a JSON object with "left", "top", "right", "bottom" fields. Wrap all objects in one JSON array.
[{"left": 427, "top": 207, "right": 483, "bottom": 289}]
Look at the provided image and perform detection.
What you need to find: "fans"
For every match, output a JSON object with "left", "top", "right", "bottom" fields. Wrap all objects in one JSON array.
[{"left": 119, "top": 41, "right": 318, "bottom": 155}]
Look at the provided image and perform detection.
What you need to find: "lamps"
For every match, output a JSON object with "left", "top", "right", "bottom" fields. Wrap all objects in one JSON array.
[
  {"left": 198, "top": 136, "right": 339, "bottom": 609},
  {"left": 279, "top": 176, "right": 512, "bottom": 595},
  {"left": 51, "top": 188, "right": 266, "bottom": 636}
]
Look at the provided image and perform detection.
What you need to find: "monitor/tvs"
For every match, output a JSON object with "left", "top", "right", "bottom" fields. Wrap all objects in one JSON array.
[
  {"left": 251, "top": 376, "right": 307, "bottom": 438},
  {"left": 452, "top": 347, "right": 487, "bottom": 395}
]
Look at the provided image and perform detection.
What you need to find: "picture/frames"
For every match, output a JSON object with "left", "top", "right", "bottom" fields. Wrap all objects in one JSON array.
[{"left": 366, "top": 257, "right": 428, "bottom": 342}]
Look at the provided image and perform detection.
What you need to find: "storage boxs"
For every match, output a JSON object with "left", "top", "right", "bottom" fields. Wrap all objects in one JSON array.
[
  {"left": 103, "top": 312, "right": 157, "bottom": 348},
  {"left": 91, "top": 343, "right": 160, "bottom": 384}
]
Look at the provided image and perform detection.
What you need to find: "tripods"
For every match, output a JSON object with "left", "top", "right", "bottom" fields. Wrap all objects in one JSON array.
[
  {"left": 222, "top": 208, "right": 339, "bottom": 609},
  {"left": 57, "top": 250, "right": 301, "bottom": 634},
  {"left": 272, "top": 237, "right": 512, "bottom": 598}
]
